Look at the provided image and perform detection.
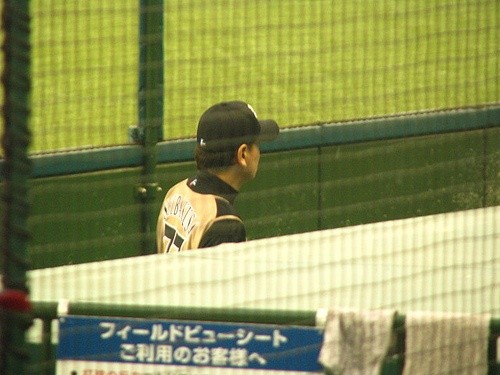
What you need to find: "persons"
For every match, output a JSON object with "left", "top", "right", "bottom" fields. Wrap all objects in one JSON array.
[{"left": 153, "top": 100, "right": 282, "bottom": 255}]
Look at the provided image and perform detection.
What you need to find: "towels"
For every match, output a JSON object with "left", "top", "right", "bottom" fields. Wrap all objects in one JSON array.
[
  {"left": 316, "top": 307, "right": 397, "bottom": 375},
  {"left": 401, "top": 309, "right": 493, "bottom": 375}
]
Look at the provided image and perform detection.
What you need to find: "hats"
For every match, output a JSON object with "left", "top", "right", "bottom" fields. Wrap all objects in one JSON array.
[{"left": 194, "top": 99, "right": 279, "bottom": 148}]
